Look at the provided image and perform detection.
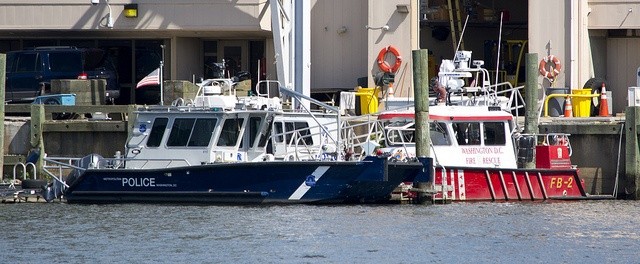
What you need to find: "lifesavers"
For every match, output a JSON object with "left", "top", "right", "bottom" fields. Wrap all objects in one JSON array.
[
  {"left": 378, "top": 48, "right": 402, "bottom": 73},
  {"left": 584, "top": 77, "right": 609, "bottom": 117},
  {"left": 540, "top": 57, "right": 561, "bottom": 78}
]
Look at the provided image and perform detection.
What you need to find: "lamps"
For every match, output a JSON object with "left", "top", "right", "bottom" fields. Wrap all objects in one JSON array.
[{"left": 396, "top": 3, "right": 411, "bottom": 14}]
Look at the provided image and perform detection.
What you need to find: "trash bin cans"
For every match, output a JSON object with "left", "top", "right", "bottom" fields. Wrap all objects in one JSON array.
[
  {"left": 546, "top": 87, "right": 570, "bottom": 116},
  {"left": 357, "top": 88, "right": 380, "bottom": 114},
  {"left": 349, "top": 90, "right": 360, "bottom": 116},
  {"left": 572, "top": 88, "right": 592, "bottom": 117}
]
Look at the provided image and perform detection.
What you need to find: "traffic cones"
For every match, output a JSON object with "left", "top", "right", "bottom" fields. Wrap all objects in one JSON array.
[
  {"left": 389, "top": 82, "right": 393, "bottom": 96},
  {"left": 563, "top": 95, "right": 574, "bottom": 117},
  {"left": 599, "top": 83, "right": 609, "bottom": 116}
]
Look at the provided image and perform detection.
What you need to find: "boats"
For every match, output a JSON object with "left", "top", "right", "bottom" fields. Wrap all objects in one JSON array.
[
  {"left": 363, "top": 10, "right": 590, "bottom": 202},
  {"left": 42, "top": 58, "right": 424, "bottom": 206}
]
